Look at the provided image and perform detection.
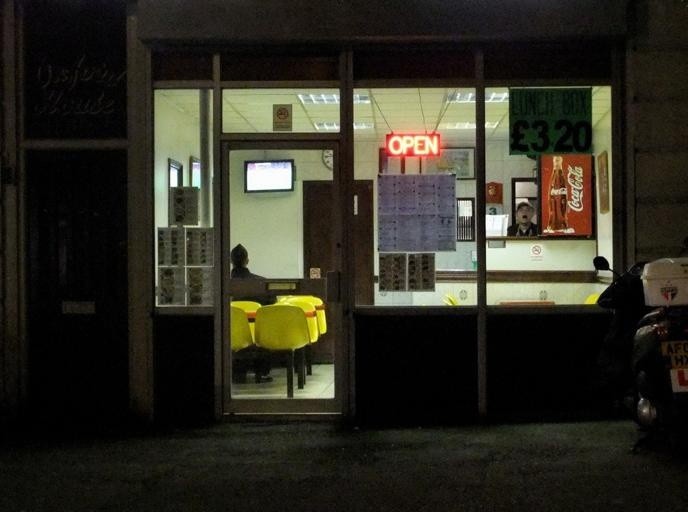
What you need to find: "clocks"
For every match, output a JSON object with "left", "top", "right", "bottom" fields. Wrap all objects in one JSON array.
[{"left": 322, "top": 149, "right": 334, "bottom": 171}]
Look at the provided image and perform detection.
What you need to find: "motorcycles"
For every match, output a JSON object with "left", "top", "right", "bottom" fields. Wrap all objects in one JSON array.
[{"left": 594, "top": 256, "right": 688, "bottom": 453}]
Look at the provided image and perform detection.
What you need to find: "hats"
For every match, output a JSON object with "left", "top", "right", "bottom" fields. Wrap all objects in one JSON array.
[{"left": 517, "top": 199, "right": 534, "bottom": 211}]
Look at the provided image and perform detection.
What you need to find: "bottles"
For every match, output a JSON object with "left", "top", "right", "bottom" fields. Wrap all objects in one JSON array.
[{"left": 546, "top": 156, "right": 569, "bottom": 232}]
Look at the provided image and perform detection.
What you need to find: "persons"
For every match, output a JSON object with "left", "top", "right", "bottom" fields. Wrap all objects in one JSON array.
[
  {"left": 506, "top": 200, "right": 537, "bottom": 236},
  {"left": 229, "top": 243, "right": 277, "bottom": 384}
]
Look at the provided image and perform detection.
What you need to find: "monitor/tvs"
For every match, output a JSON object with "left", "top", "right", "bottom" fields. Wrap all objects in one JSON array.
[{"left": 244, "top": 159, "right": 294, "bottom": 193}]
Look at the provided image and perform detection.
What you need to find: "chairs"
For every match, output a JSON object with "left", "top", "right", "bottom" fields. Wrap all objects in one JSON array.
[{"left": 229, "top": 295, "right": 328, "bottom": 398}]
